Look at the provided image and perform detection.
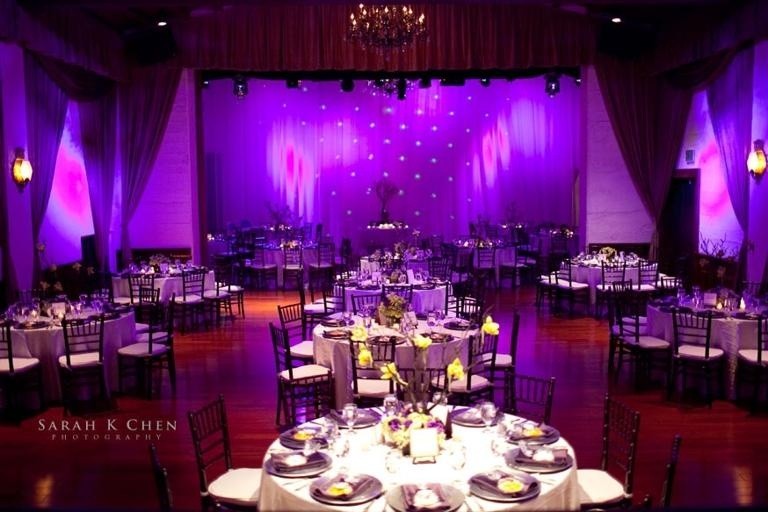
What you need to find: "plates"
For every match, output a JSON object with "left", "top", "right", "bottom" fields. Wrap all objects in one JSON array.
[
  {"left": 13, "top": 289, "right": 128, "bottom": 329},
  {"left": 650, "top": 285, "right": 765, "bottom": 321},
  {"left": 337, "top": 279, "right": 449, "bottom": 292},
  {"left": 321, "top": 315, "right": 480, "bottom": 345},
  {"left": 264, "top": 393, "right": 573, "bottom": 511}
]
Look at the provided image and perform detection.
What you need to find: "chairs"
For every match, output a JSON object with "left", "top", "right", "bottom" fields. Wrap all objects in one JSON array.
[{"left": 0, "top": 222, "right": 768, "bottom": 510}]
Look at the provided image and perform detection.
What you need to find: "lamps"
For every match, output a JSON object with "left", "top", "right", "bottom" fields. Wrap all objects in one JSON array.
[
  {"left": 13, "top": 147, "right": 34, "bottom": 189},
  {"left": 343, "top": 1, "right": 429, "bottom": 59},
  {"left": 744, "top": 138, "right": 767, "bottom": 181}
]
